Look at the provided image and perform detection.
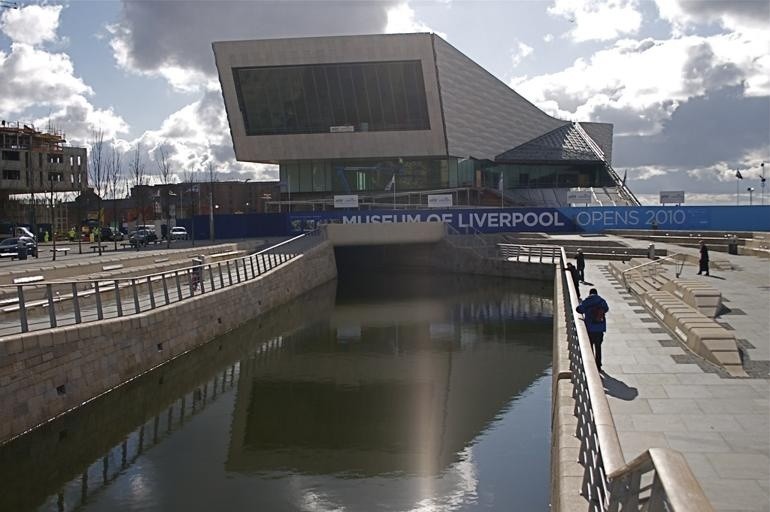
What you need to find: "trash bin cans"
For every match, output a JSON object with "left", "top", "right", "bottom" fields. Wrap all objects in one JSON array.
[
  {"left": 17, "top": 241, "right": 27, "bottom": 260},
  {"left": 32, "top": 245, "right": 36, "bottom": 257},
  {"left": 729, "top": 243, "right": 738, "bottom": 254}
]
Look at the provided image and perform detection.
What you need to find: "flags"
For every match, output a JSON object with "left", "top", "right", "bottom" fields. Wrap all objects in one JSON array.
[
  {"left": 735, "top": 171, "right": 743, "bottom": 179},
  {"left": 384, "top": 175, "right": 394, "bottom": 191},
  {"left": 498, "top": 172, "right": 503, "bottom": 193},
  {"left": 277, "top": 180, "right": 288, "bottom": 187}
]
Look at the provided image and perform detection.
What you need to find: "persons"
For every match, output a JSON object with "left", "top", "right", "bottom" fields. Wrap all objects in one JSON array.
[
  {"left": 697, "top": 240, "right": 709, "bottom": 276},
  {"left": 576, "top": 289, "right": 609, "bottom": 372},
  {"left": 68, "top": 227, "right": 75, "bottom": 242},
  {"left": 563, "top": 262, "right": 580, "bottom": 299},
  {"left": 574, "top": 248, "right": 585, "bottom": 281}
]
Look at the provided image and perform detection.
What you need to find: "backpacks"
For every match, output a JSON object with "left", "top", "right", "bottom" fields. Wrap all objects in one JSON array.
[{"left": 587, "top": 304, "right": 604, "bottom": 323}]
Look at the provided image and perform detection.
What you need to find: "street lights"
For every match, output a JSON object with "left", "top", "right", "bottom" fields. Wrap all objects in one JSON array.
[{"left": 747, "top": 186, "right": 754, "bottom": 206}]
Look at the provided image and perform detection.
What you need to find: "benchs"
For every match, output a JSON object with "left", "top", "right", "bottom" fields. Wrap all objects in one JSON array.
[{"left": 642, "top": 275, "right": 750, "bottom": 379}]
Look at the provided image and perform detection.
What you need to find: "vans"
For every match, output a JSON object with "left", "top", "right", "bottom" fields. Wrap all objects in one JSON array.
[
  {"left": 101, "top": 226, "right": 124, "bottom": 241},
  {"left": 128, "top": 224, "right": 155, "bottom": 245}
]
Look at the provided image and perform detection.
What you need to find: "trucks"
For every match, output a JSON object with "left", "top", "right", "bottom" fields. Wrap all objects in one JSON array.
[{"left": 0, "top": 223, "right": 36, "bottom": 239}]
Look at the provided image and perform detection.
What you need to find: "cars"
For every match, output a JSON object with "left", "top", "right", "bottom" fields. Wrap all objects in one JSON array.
[
  {"left": 169, "top": 225, "right": 189, "bottom": 241},
  {"left": 0, "top": 237, "right": 37, "bottom": 260}
]
